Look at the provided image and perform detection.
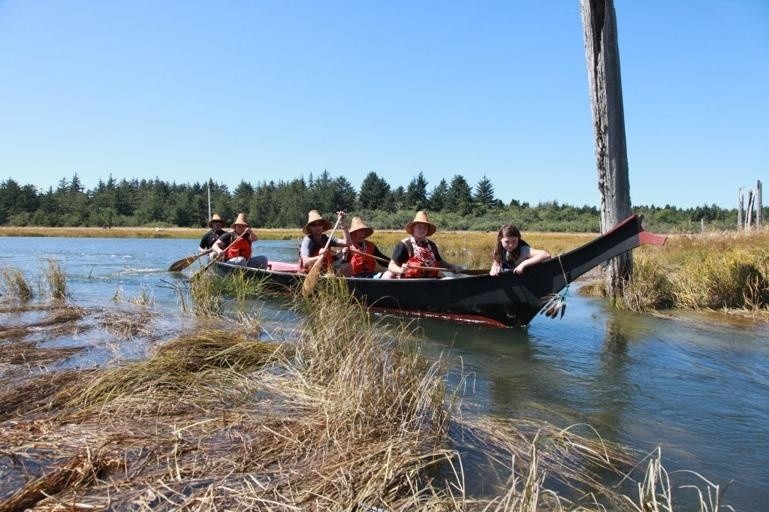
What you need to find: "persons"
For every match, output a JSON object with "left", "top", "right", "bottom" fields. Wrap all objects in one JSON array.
[
  {"left": 297, "top": 209, "right": 352, "bottom": 275},
  {"left": 387, "top": 210, "right": 463, "bottom": 278},
  {"left": 198, "top": 212, "right": 232, "bottom": 262},
  {"left": 336, "top": 216, "right": 400, "bottom": 279},
  {"left": 212, "top": 212, "right": 268, "bottom": 270},
  {"left": 489, "top": 223, "right": 551, "bottom": 277}
]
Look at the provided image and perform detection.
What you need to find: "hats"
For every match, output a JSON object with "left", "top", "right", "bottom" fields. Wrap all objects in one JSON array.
[
  {"left": 303, "top": 210, "right": 332, "bottom": 234},
  {"left": 209, "top": 214, "right": 225, "bottom": 228},
  {"left": 231, "top": 213, "right": 249, "bottom": 229},
  {"left": 349, "top": 217, "right": 373, "bottom": 238},
  {"left": 406, "top": 211, "right": 436, "bottom": 236}
]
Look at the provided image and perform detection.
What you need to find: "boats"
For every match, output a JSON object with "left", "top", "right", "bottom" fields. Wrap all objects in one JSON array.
[{"left": 199, "top": 213, "right": 666, "bottom": 332}]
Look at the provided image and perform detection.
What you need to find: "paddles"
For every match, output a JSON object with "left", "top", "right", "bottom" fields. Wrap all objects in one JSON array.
[
  {"left": 186, "top": 231, "right": 247, "bottom": 283},
  {"left": 168, "top": 248, "right": 212, "bottom": 271},
  {"left": 302, "top": 211, "right": 343, "bottom": 298}
]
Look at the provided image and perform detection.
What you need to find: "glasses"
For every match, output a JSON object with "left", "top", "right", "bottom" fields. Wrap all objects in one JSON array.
[{"left": 309, "top": 222, "right": 324, "bottom": 227}]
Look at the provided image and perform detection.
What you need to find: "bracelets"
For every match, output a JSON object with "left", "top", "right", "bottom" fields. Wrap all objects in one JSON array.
[{"left": 341, "top": 226, "right": 347, "bottom": 230}]
